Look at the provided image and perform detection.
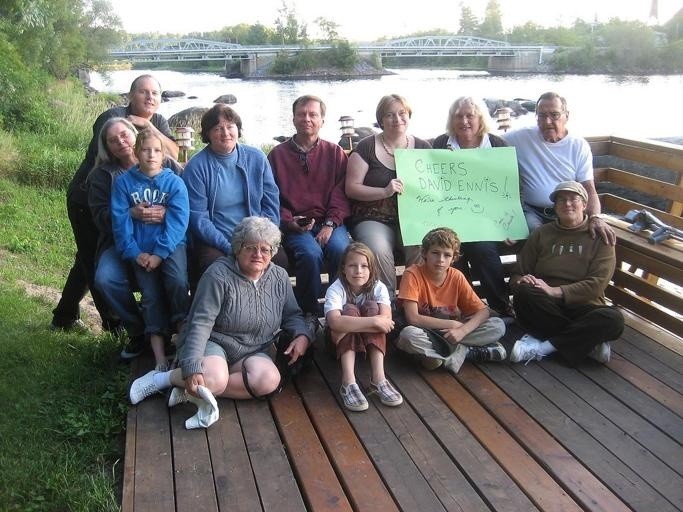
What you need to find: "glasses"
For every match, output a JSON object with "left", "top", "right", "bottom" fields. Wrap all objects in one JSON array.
[
  {"left": 534, "top": 112, "right": 568, "bottom": 121},
  {"left": 240, "top": 244, "right": 276, "bottom": 257}
]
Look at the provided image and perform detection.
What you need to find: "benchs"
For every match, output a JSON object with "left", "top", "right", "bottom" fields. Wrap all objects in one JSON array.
[{"left": 139, "top": 137, "right": 683, "bottom": 338}]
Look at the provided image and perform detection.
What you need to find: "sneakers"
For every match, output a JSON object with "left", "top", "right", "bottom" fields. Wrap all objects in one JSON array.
[
  {"left": 339, "top": 381, "right": 371, "bottom": 412},
  {"left": 303, "top": 312, "right": 322, "bottom": 334},
  {"left": 128, "top": 356, "right": 193, "bottom": 407},
  {"left": 510, "top": 331, "right": 544, "bottom": 364},
  {"left": 369, "top": 376, "right": 405, "bottom": 407},
  {"left": 587, "top": 340, "right": 612, "bottom": 364},
  {"left": 119, "top": 335, "right": 180, "bottom": 360},
  {"left": 48, "top": 314, "right": 93, "bottom": 338},
  {"left": 465, "top": 341, "right": 509, "bottom": 362},
  {"left": 493, "top": 304, "right": 516, "bottom": 326},
  {"left": 423, "top": 355, "right": 443, "bottom": 370}
]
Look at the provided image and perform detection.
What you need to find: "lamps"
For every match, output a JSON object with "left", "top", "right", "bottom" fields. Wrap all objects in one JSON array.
[
  {"left": 337, "top": 112, "right": 361, "bottom": 150},
  {"left": 496, "top": 104, "right": 513, "bottom": 133},
  {"left": 173, "top": 125, "right": 197, "bottom": 162}
]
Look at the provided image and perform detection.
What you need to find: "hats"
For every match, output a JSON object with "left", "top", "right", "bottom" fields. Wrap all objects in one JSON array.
[{"left": 548, "top": 179, "right": 589, "bottom": 205}]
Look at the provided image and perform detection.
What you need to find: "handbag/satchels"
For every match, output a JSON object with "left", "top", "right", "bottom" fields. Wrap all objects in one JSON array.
[{"left": 279, "top": 343, "right": 313, "bottom": 379}]
